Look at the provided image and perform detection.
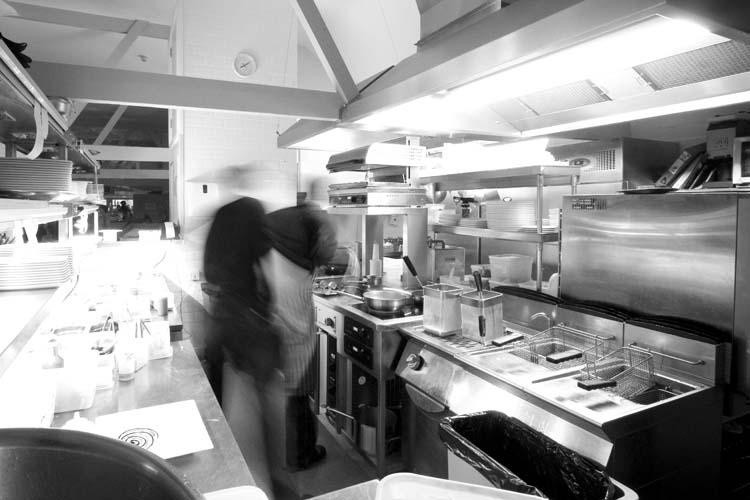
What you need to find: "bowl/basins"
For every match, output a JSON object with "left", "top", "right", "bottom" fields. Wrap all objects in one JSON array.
[{"left": 72, "top": 180, "right": 89, "bottom": 195}]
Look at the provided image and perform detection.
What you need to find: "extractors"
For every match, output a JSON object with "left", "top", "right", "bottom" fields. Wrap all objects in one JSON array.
[{"left": 277, "top": 0, "right": 750, "bottom": 151}]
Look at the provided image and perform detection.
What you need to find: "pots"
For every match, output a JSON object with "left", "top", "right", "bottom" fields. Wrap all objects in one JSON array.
[
  {"left": 412, "top": 290, "right": 424, "bottom": 304},
  {"left": 353, "top": 402, "right": 398, "bottom": 455},
  {"left": 47, "top": 94, "right": 72, "bottom": 116},
  {"left": 331, "top": 288, "right": 413, "bottom": 315},
  {"left": 357, "top": 274, "right": 382, "bottom": 286},
  {"left": 0, "top": 428, "right": 205, "bottom": 500}
]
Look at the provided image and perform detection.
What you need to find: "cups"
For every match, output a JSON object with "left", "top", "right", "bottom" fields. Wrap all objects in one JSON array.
[
  {"left": 117, "top": 353, "right": 136, "bottom": 383},
  {"left": 158, "top": 297, "right": 169, "bottom": 317}
]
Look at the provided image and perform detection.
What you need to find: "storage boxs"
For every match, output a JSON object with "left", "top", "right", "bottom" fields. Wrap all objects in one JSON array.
[{"left": 489, "top": 254, "right": 533, "bottom": 285}]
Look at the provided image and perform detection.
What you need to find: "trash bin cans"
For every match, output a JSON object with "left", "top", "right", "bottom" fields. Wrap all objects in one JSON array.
[{"left": 439, "top": 408, "right": 640, "bottom": 500}]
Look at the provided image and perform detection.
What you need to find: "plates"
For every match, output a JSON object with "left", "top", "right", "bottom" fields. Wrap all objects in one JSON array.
[
  {"left": 85, "top": 183, "right": 105, "bottom": 200},
  {"left": 437, "top": 198, "right": 559, "bottom": 234},
  {"left": 0, "top": 243, "right": 76, "bottom": 292},
  {"left": 0, "top": 154, "right": 74, "bottom": 201},
  {"left": 616, "top": 186, "right": 680, "bottom": 196},
  {"left": 99, "top": 399, "right": 214, "bottom": 463}
]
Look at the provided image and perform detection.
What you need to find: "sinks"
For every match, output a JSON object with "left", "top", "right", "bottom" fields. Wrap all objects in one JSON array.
[
  {"left": 573, "top": 364, "right": 701, "bottom": 405},
  {"left": 508, "top": 343, "right": 602, "bottom": 372}
]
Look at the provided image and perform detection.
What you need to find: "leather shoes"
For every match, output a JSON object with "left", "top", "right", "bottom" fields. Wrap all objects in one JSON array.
[{"left": 287, "top": 446, "right": 325, "bottom": 473}]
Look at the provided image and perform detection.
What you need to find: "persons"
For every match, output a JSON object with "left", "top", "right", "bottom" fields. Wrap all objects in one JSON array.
[
  {"left": 258, "top": 176, "right": 360, "bottom": 470},
  {"left": 119, "top": 201, "right": 132, "bottom": 221},
  {"left": 197, "top": 162, "right": 314, "bottom": 500}
]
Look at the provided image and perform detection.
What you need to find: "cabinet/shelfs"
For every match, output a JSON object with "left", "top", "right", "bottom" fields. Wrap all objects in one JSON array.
[
  {"left": 0, "top": 39, "right": 106, "bottom": 377},
  {"left": 420, "top": 167, "right": 582, "bottom": 294}
]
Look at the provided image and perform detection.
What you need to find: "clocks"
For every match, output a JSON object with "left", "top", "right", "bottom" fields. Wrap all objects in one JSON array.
[{"left": 232, "top": 53, "right": 256, "bottom": 78}]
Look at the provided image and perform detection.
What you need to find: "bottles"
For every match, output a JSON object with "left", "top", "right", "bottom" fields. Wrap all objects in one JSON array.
[{"left": 61, "top": 412, "right": 95, "bottom": 435}]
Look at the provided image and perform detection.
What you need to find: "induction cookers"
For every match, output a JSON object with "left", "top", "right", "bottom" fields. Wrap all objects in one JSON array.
[
  {"left": 338, "top": 284, "right": 411, "bottom": 300},
  {"left": 346, "top": 290, "right": 424, "bottom": 321}
]
